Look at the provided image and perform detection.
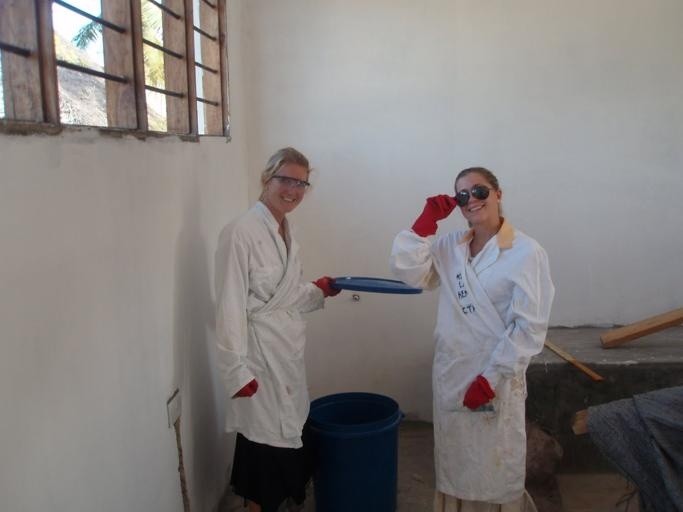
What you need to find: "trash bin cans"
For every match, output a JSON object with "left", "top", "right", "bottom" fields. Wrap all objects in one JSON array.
[{"left": 302, "top": 392, "right": 405, "bottom": 512}]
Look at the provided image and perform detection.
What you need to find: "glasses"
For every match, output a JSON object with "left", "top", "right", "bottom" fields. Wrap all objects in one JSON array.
[
  {"left": 454, "top": 185, "right": 498, "bottom": 207},
  {"left": 266, "top": 173, "right": 312, "bottom": 194}
]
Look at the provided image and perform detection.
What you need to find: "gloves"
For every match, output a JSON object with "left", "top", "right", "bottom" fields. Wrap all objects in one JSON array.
[
  {"left": 233, "top": 378, "right": 258, "bottom": 399},
  {"left": 462, "top": 374, "right": 496, "bottom": 411},
  {"left": 310, "top": 274, "right": 343, "bottom": 298},
  {"left": 411, "top": 194, "right": 457, "bottom": 238}
]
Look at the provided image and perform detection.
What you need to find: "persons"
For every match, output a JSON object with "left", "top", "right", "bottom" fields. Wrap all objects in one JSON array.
[
  {"left": 388, "top": 166, "right": 556, "bottom": 512},
  {"left": 202, "top": 146, "right": 345, "bottom": 511}
]
